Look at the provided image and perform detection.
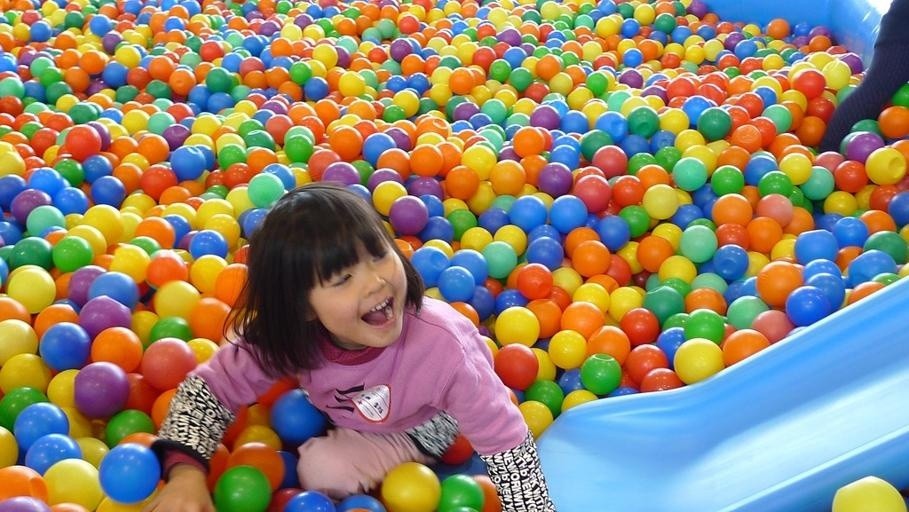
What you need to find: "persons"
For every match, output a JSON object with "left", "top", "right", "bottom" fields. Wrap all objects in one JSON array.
[
  {"left": 148, "top": 182, "right": 556, "bottom": 512},
  {"left": 819, "top": 0, "right": 909, "bottom": 153}
]
